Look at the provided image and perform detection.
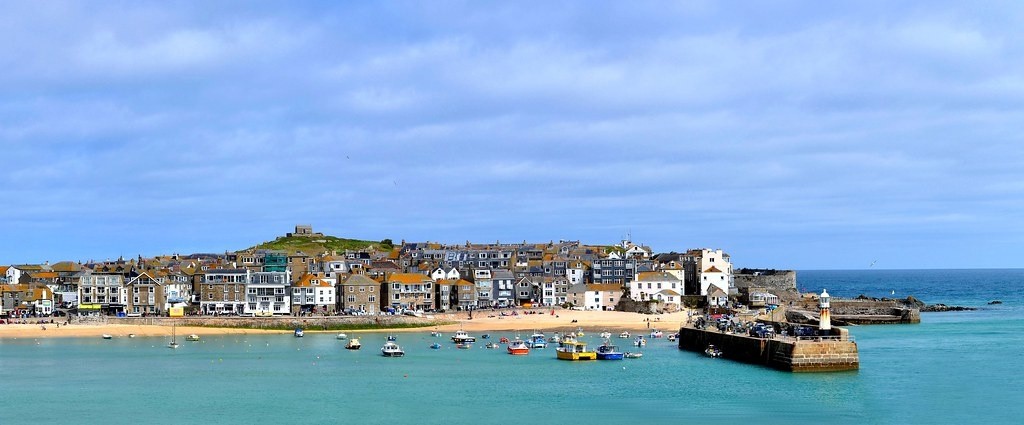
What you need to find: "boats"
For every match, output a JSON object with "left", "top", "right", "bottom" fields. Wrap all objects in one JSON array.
[
  {"left": 430, "top": 342, "right": 442, "bottom": 350},
  {"left": 667, "top": 334, "right": 676, "bottom": 342},
  {"left": 345, "top": 338, "right": 361, "bottom": 350},
  {"left": 523, "top": 330, "right": 547, "bottom": 348},
  {"left": 634, "top": 335, "right": 646, "bottom": 348},
  {"left": 451, "top": 329, "right": 476, "bottom": 343},
  {"left": 129, "top": 333, "right": 136, "bottom": 338},
  {"left": 294, "top": 327, "right": 303, "bottom": 337},
  {"left": 485, "top": 341, "right": 499, "bottom": 349},
  {"left": 620, "top": 331, "right": 631, "bottom": 338},
  {"left": 675, "top": 332, "right": 680, "bottom": 338},
  {"left": 594, "top": 335, "right": 623, "bottom": 360},
  {"left": 482, "top": 334, "right": 490, "bottom": 339},
  {"left": 554, "top": 334, "right": 598, "bottom": 360},
  {"left": 456, "top": 344, "right": 472, "bottom": 350},
  {"left": 547, "top": 336, "right": 560, "bottom": 343},
  {"left": 499, "top": 336, "right": 510, "bottom": 344},
  {"left": 576, "top": 327, "right": 584, "bottom": 336},
  {"left": 598, "top": 328, "right": 611, "bottom": 338},
  {"left": 705, "top": 344, "right": 723, "bottom": 358},
  {"left": 432, "top": 328, "right": 442, "bottom": 338},
  {"left": 649, "top": 328, "right": 664, "bottom": 338},
  {"left": 623, "top": 350, "right": 643, "bottom": 358},
  {"left": 337, "top": 333, "right": 348, "bottom": 340},
  {"left": 103, "top": 334, "right": 112, "bottom": 339},
  {"left": 380, "top": 335, "right": 405, "bottom": 357},
  {"left": 186, "top": 334, "right": 200, "bottom": 341},
  {"left": 507, "top": 330, "right": 529, "bottom": 355}
]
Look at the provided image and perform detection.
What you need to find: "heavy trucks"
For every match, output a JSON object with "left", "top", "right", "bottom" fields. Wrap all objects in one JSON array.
[{"left": 67, "top": 300, "right": 78, "bottom": 309}]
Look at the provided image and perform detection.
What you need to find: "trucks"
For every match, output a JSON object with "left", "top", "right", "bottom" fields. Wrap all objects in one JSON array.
[{"left": 344, "top": 308, "right": 355, "bottom": 314}]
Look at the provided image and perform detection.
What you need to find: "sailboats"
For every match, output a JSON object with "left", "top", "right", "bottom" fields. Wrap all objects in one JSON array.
[{"left": 168, "top": 318, "right": 179, "bottom": 348}]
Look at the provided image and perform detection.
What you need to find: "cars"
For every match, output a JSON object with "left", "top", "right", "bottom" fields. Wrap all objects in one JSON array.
[
  {"left": 53, "top": 311, "right": 66, "bottom": 317},
  {"left": 500, "top": 302, "right": 507, "bottom": 308},
  {"left": 714, "top": 316, "right": 814, "bottom": 339},
  {"left": 384, "top": 306, "right": 395, "bottom": 315},
  {"left": 128, "top": 312, "right": 142, "bottom": 317},
  {"left": 60, "top": 301, "right": 67, "bottom": 308},
  {"left": 401, "top": 308, "right": 414, "bottom": 315}
]
